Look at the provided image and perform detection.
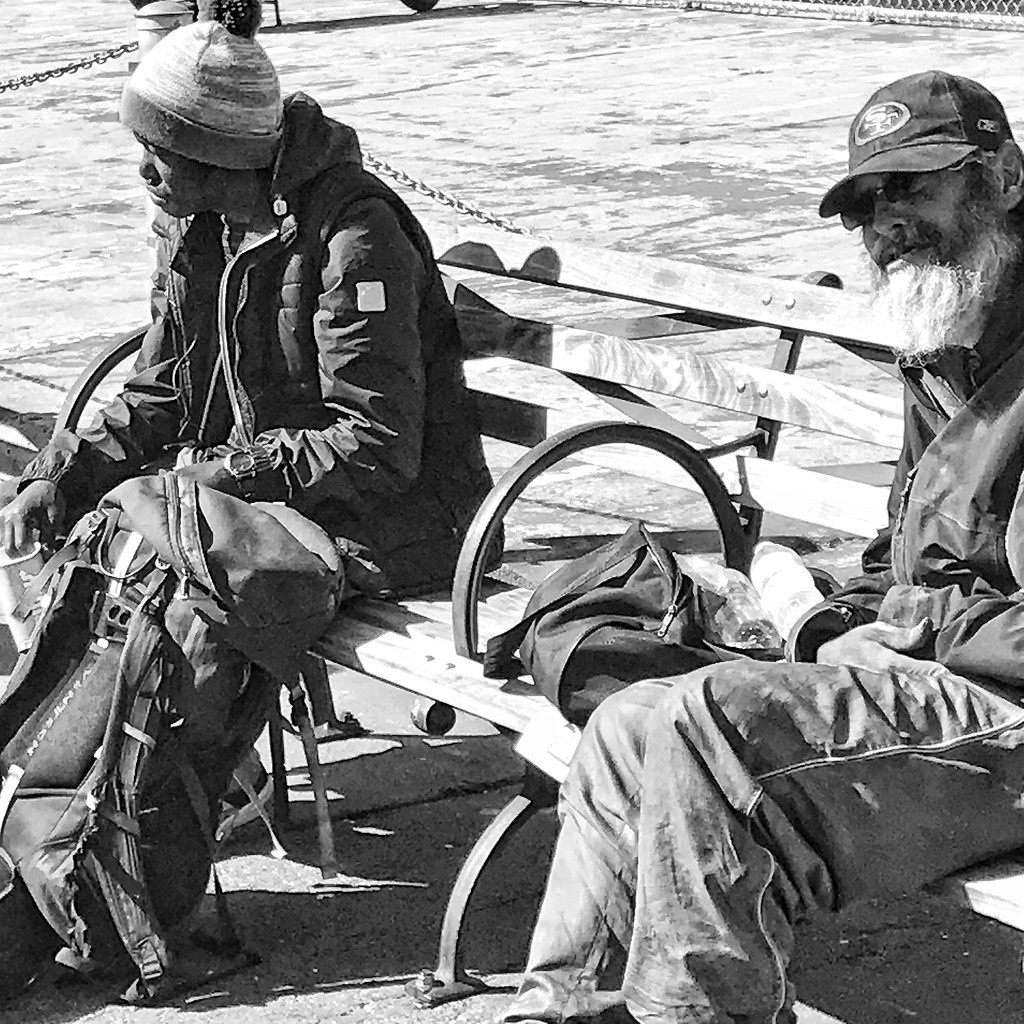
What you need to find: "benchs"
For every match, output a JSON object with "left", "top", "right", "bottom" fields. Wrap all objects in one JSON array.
[{"left": 53, "top": 217, "right": 1024, "bottom": 1010}]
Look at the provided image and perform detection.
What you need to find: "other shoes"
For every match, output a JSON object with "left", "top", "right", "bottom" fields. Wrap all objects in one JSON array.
[{"left": 216, "top": 763, "right": 274, "bottom": 840}]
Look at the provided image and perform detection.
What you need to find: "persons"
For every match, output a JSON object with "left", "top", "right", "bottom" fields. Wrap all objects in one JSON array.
[
  {"left": 504, "top": 71, "right": 1024, "bottom": 1024},
  {"left": 0, "top": 19, "right": 505, "bottom": 1002}
]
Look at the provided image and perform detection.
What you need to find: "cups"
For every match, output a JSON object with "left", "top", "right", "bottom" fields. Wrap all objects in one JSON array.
[{"left": 0, "top": 542, "right": 51, "bottom": 653}]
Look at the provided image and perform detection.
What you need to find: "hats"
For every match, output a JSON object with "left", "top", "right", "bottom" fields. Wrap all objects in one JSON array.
[
  {"left": 820, "top": 69, "right": 1013, "bottom": 218},
  {"left": 118, "top": 0, "right": 284, "bottom": 172}
]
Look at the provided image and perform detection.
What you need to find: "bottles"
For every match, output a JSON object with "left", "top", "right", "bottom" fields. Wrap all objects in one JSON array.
[
  {"left": 750, "top": 540, "right": 827, "bottom": 639},
  {"left": 705, "top": 566, "right": 782, "bottom": 650}
]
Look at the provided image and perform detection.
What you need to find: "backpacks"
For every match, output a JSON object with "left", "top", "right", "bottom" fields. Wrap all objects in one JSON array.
[{"left": 0, "top": 471, "right": 345, "bottom": 997}]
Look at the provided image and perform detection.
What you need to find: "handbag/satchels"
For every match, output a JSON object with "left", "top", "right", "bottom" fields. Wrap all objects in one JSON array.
[{"left": 485, "top": 518, "right": 845, "bottom": 728}]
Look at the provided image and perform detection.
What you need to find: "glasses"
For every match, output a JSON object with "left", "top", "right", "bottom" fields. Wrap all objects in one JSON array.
[{"left": 840, "top": 151, "right": 992, "bottom": 230}]
